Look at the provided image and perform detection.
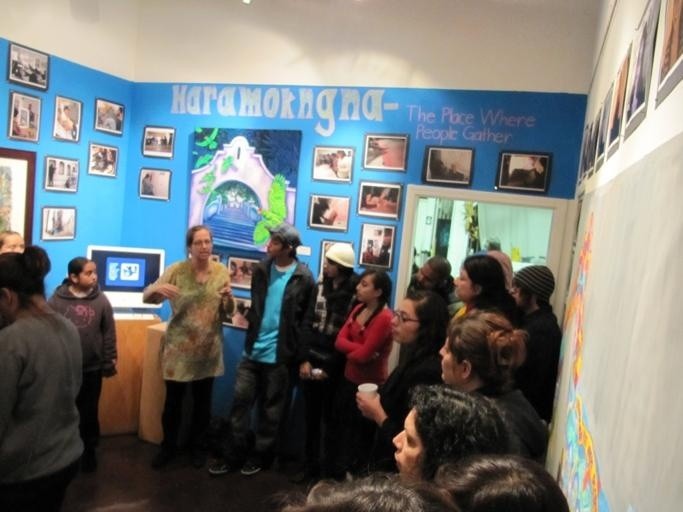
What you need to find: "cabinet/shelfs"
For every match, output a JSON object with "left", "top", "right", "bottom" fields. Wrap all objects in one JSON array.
[{"left": 97, "top": 312, "right": 163, "bottom": 438}]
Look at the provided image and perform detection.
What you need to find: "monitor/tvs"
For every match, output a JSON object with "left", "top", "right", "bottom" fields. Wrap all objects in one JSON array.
[{"left": 87, "top": 246, "right": 165, "bottom": 322}]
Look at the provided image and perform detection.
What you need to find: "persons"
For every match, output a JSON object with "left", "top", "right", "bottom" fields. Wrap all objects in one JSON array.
[
  {"left": 482, "top": 250, "right": 512, "bottom": 291},
  {"left": 438, "top": 456, "right": 572, "bottom": 512},
  {"left": 0, "top": 229, "right": 25, "bottom": 255},
  {"left": 361, "top": 225, "right": 390, "bottom": 267},
  {"left": 313, "top": 150, "right": 353, "bottom": 180},
  {"left": 98, "top": 103, "right": 123, "bottom": 132},
  {"left": 508, "top": 263, "right": 564, "bottom": 426},
  {"left": 47, "top": 255, "right": 117, "bottom": 475},
  {"left": 253, "top": 470, "right": 462, "bottom": 511},
  {"left": 239, "top": 261, "right": 247, "bottom": 274},
  {"left": 12, "top": 102, "right": 36, "bottom": 137},
  {"left": 57, "top": 103, "right": 78, "bottom": 142},
  {"left": 452, "top": 254, "right": 525, "bottom": 329},
  {"left": 0, "top": 245, "right": 83, "bottom": 512},
  {"left": 143, "top": 226, "right": 237, "bottom": 470},
  {"left": 322, "top": 266, "right": 395, "bottom": 483},
  {"left": 12, "top": 58, "right": 48, "bottom": 85},
  {"left": 355, "top": 288, "right": 450, "bottom": 476},
  {"left": 46, "top": 158, "right": 77, "bottom": 190},
  {"left": 393, "top": 382, "right": 518, "bottom": 479},
  {"left": 291, "top": 242, "right": 359, "bottom": 488},
  {"left": 208, "top": 222, "right": 316, "bottom": 476},
  {"left": 438, "top": 312, "right": 549, "bottom": 464},
  {"left": 142, "top": 170, "right": 154, "bottom": 195},
  {"left": 92, "top": 146, "right": 117, "bottom": 175},
  {"left": 406, "top": 256, "right": 465, "bottom": 316}
]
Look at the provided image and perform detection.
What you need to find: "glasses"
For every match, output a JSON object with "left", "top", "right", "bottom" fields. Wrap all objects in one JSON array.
[{"left": 390, "top": 311, "right": 421, "bottom": 323}]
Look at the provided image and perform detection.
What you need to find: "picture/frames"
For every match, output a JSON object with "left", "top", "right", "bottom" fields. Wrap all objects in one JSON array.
[{"left": 0, "top": 147, "right": 35, "bottom": 247}]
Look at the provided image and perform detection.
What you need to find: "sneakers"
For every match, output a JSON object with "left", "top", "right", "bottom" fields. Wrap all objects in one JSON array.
[
  {"left": 241, "top": 461, "right": 260, "bottom": 475},
  {"left": 82, "top": 445, "right": 97, "bottom": 472},
  {"left": 209, "top": 461, "right": 232, "bottom": 473}
]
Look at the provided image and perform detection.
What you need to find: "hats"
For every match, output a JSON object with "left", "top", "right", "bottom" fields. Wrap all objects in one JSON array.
[
  {"left": 489, "top": 250, "right": 514, "bottom": 288},
  {"left": 265, "top": 222, "right": 300, "bottom": 247},
  {"left": 326, "top": 243, "right": 355, "bottom": 267},
  {"left": 514, "top": 265, "right": 555, "bottom": 298}
]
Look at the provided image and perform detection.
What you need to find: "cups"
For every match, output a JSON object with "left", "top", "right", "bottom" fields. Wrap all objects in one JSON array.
[{"left": 358, "top": 383, "right": 379, "bottom": 397}]
